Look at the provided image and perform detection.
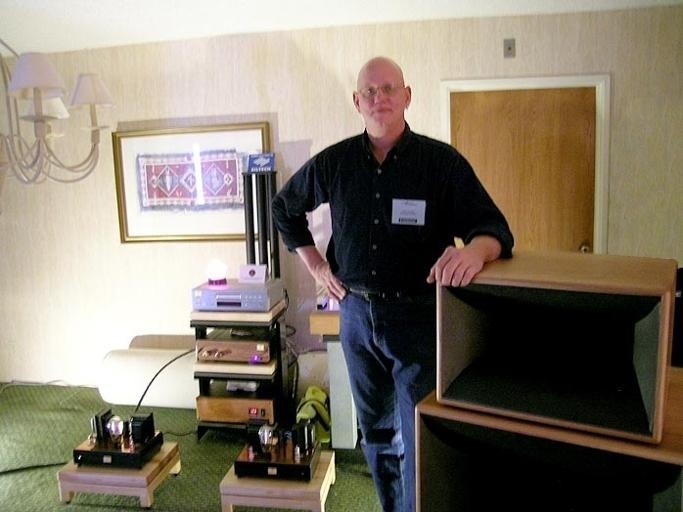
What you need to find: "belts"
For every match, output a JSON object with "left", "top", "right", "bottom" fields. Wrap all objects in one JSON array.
[{"left": 339, "top": 283, "right": 434, "bottom": 304}]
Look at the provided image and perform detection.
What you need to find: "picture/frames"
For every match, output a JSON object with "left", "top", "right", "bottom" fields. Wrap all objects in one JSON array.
[{"left": 112, "top": 121, "right": 270, "bottom": 244}]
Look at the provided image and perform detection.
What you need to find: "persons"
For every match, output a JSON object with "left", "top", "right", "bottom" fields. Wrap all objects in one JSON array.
[{"left": 269, "top": 55, "right": 514, "bottom": 512}]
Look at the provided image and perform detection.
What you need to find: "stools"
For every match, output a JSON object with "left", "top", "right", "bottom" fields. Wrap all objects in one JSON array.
[
  {"left": 57, "top": 441, "right": 182, "bottom": 508},
  {"left": 219, "top": 451, "right": 336, "bottom": 512}
]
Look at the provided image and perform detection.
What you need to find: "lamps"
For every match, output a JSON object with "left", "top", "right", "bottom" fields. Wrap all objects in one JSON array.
[{"left": 0, "top": 36, "right": 114, "bottom": 186}]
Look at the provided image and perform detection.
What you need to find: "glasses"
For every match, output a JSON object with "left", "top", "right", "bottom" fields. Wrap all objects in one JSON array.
[{"left": 356, "top": 82, "right": 406, "bottom": 98}]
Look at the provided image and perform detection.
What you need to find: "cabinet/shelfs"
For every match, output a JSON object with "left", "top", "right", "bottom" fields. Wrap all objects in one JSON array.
[{"left": 190, "top": 305, "right": 290, "bottom": 440}]
